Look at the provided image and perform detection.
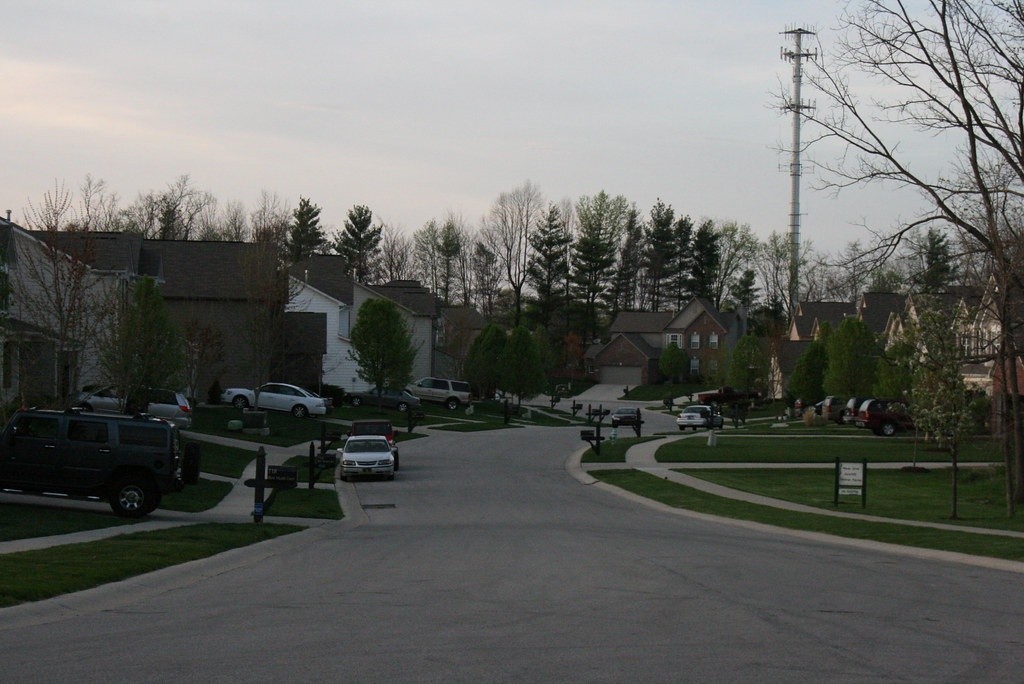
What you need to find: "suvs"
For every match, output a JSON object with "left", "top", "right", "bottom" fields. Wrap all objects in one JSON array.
[
  {"left": 0, "top": 404, "right": 201, "bottom": 518},
  {"left": 402, "top": 377, "right": 474, "bottom": 411},
  {"left": 73, "top": 383, "right": 192, "bottom": 429},
  {"left": 842, "top": 396, "right": 870, "bottom": 423},
  {"left": 822, "top": 395, "right": 847, "bottom": 425},
  {"left": 347, "top": 418, "right": 400, "bottom": 470},
  {"left": 855, "top": 398, "right": 919, "bottom": 436}
]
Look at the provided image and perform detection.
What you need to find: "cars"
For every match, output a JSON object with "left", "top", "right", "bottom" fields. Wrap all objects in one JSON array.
[
  {"left": 611, "top": 408, "right": 641, "bottom": 428},
  {"left": 345, "top": 386, "right": 421, "bottom": 414},
  {"left": 337, "top": 436, "right": 398, "bottom": 481},
  {"left": 794, "top": 398, "right": 823, "bottom": 419},
  {"left": 675, "top": 405, "right": 724, "bottom": 430},
  {"left": 218, "top": 382, "right": 327, "bottom": 418}
]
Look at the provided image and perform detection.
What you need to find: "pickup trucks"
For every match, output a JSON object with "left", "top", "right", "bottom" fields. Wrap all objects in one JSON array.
[{"left": 697, "top": 386, "right": 762, "bottom": 407}]
